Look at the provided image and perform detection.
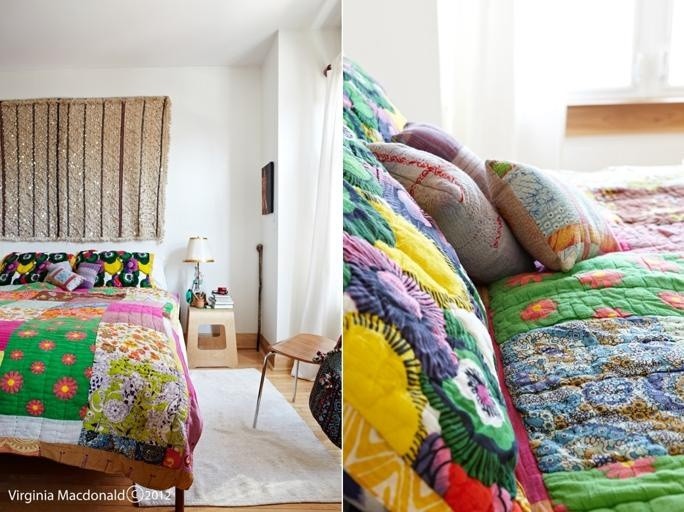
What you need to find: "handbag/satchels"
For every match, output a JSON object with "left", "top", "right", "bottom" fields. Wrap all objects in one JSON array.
[{"left": 309, "top": 350, "right": 342, "bottom": 449}]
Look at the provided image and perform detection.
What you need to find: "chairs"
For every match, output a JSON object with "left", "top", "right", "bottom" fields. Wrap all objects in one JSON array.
[{"left": 249, "top": 333, "right": 342, "bottom": 432}]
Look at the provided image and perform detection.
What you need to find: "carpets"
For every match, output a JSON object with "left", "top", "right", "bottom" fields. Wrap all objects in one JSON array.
[{"left": 135, "top": 368, "right": 342, "bottom": 507}]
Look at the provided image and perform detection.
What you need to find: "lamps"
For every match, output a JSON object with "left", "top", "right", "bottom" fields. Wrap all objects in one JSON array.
[{"left": 182, "top": 235, "right": 214, "bottom": 306}]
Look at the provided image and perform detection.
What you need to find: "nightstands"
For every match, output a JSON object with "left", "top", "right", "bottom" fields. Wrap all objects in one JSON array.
[{"left": 186, "top": 307, "right": 239, "bottom": 368}]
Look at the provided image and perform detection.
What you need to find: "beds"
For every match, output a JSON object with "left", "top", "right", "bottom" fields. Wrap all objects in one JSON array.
[
  {"left": 0, "top": 238, "right": 202, "bottom": 510},
  {"left": 342, "top": 59, "right": 684, "bottom": 510}
]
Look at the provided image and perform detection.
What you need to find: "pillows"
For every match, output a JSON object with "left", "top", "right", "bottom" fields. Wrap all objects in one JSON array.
[
  {"left": 393, "top": 121, "right": 493, "bottom": 199},
  {"left": 368, "top": 142, "right": 526, "bottom": 285},
  {"left": 482, "top": 159, "right": 631, "bottom": 274},
  {"left": 0, "top": 255, "right": 75, "bottom": 293},
  {"left": 74, "top": 250, "right": 153, "bottom": 288}
]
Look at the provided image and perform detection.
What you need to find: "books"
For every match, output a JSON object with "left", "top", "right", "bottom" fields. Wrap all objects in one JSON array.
[{"left": 208, "top": 290, "right": 234, "bottom": 308}]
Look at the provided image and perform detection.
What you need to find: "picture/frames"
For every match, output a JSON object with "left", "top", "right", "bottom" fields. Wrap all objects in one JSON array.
[{"left": 260, "top": 160, "right": 275, "bottom": 216}]
